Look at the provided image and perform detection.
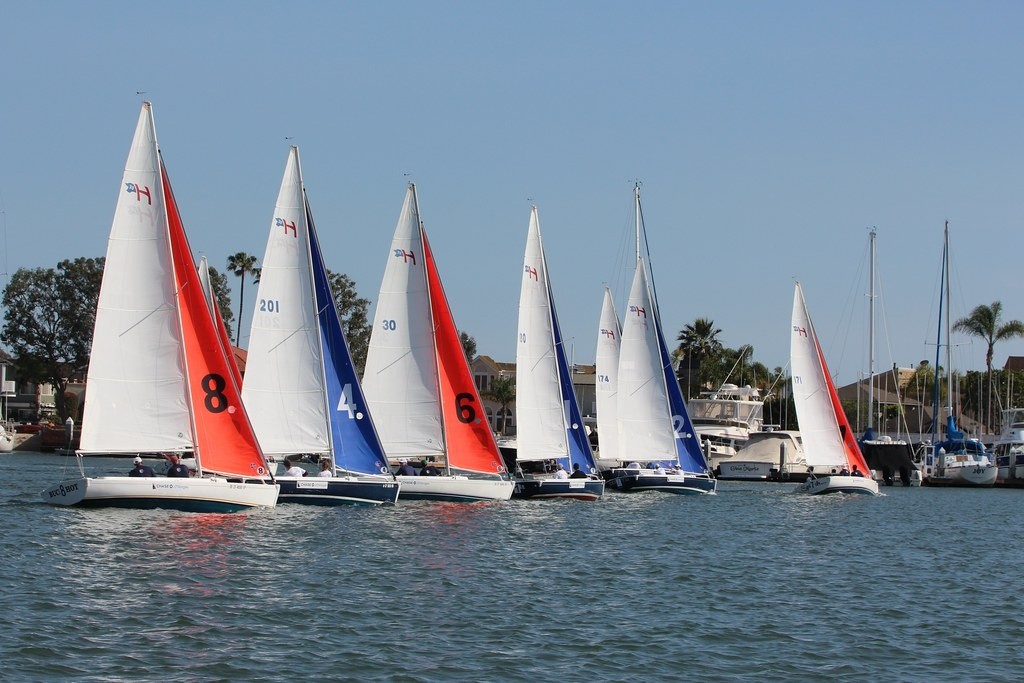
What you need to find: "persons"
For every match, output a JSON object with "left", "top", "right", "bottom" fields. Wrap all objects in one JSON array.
[
  {"left": 674, "top": 465, "right": 685, "bottom": 476},
  {"left": 554, "top": 464, "right": 569, "bottom": 479},
  {"left": 168, "top": 456, "right": 189, "bottom": 478},
  {"left": 316, "top": 461, "right": 333, "bottom": 477},
  {"left": 267, "top": 456, "right": 275, "bottom": 463},
  {"left": 851, "top": 464, "right": 863, "bottom": 477},
  {"left": 570, "top": 463, "right": 588, "bottom": 478},
  {"left": 840, "top": 464, "right": 850, "bottom": 476},
  {"left": 283, "top": 459, "right": 308, "bottom": 477},
  {"left": 654, "top": 464, "right": 666, "bottom": 475},
  {"left": 420, "top": 460, "right": 443, "bottom": 476},
  {"left": 128, "top": 457, "right": 156, "bottom": 477},
  {"left": 395, "top": 458, "right": 419, "bottom": 476}
]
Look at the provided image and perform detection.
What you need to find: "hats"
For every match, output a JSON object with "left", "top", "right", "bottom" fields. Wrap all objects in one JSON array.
[
  {"left": 134, "top": 457, "right": 142, "bottom": 464},
  {"left": 675, "top": 464, "right": 681, "bottom": 467},
  {"left": 557, "top": 463, "right": 563, "bottom": 469},
  {"left": 656, "top": 464, "right": 659, "bottom": 467},
  {"left": 422, "top": 460, "right": 427, "bottom": 465},
  {"left": 573, "top": 463, "right": 579, "bottom": 470},
  {"left": 284, "top": 460, "right": 291, "bottom": 466},
  {"left": 171, "top": 456, "right": 178, "bottom": 462},
  {"left": 399, "top": 458, "right": 407, "bottom": 463}
]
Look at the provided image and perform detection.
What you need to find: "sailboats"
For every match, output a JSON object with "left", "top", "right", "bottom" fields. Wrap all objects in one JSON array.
[
  {"left": 790, "top": 281, "right": 879, "bottom": 497},
  {"left": 40, "top": 101, "right": 281, "bottom": 513},
  {"left": 856, "top": 229, "right": 924, "bottom": 487},
  {"left": 335, "top": 181, "right": 516, "bottom": 504},
  {"left": 196, "top": 145, "right": 402, "bottom": 507},
  {"left": 591, "top": 257, "right": 717, "bottom": 496},
  {"left": 912, "top": 221, "right": 998, "bottom": 485},
  {"left": 514, "top": 206, "right": 606, "bottom": 501}
]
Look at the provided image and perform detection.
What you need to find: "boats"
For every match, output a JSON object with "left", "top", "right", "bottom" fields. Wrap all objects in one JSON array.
[
  {"left": 686, "top": 384, "right": 840, "bottom": 480},
  {"left": 154, "top": 452, "right": 278, "bottom": 476},
  {"left": 0, "top": 419, "right": 15, "bottom": 452},
  {"left": 988, "top": 408, "right": 1024, "bottom": 486},
  {"left": 495, "top": 436, "right": 518, "bottom": 472}
]
